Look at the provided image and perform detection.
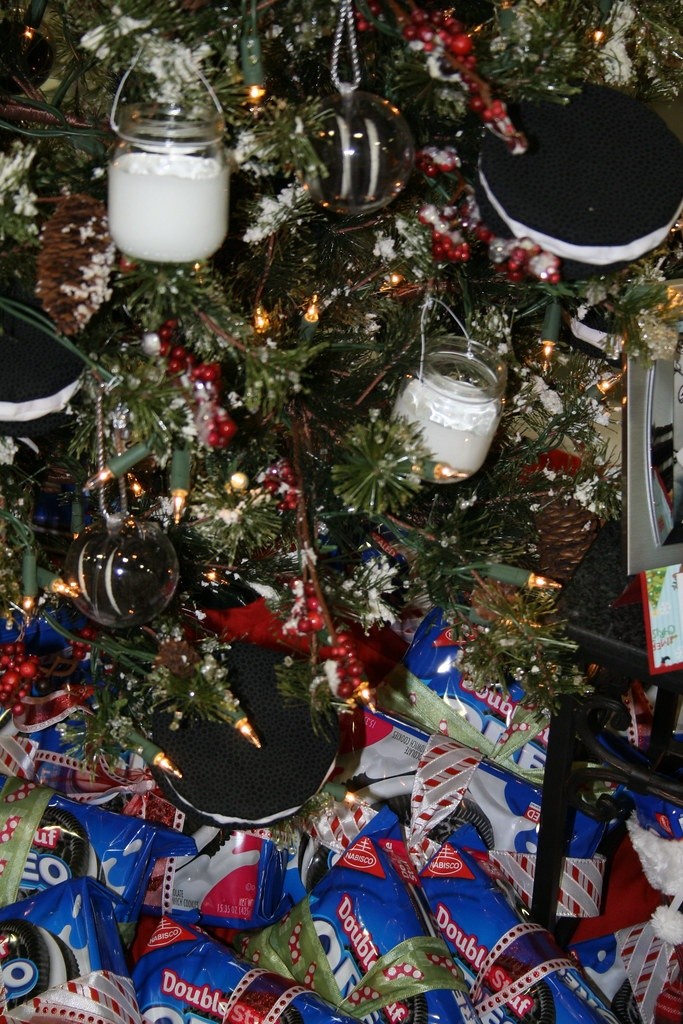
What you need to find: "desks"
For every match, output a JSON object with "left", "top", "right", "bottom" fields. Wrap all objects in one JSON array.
[{"left": 529, "top": 516, "right": 683, "bottom": 946}]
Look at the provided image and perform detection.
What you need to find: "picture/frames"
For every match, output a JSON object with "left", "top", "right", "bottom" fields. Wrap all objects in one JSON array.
[
  {"left": 640, "top": 563, "right": 683, "bottom": 675},
  {"left": 626, "top": 353, "right": 683, "bottom": 575}
]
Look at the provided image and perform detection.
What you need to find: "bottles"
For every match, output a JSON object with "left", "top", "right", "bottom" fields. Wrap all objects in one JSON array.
[
  {"left": 105, "top": 101, "right": 230, "bottom": 262},
  {"left": 392, "top": 336, "right": 508, "bottom": 485}
]
